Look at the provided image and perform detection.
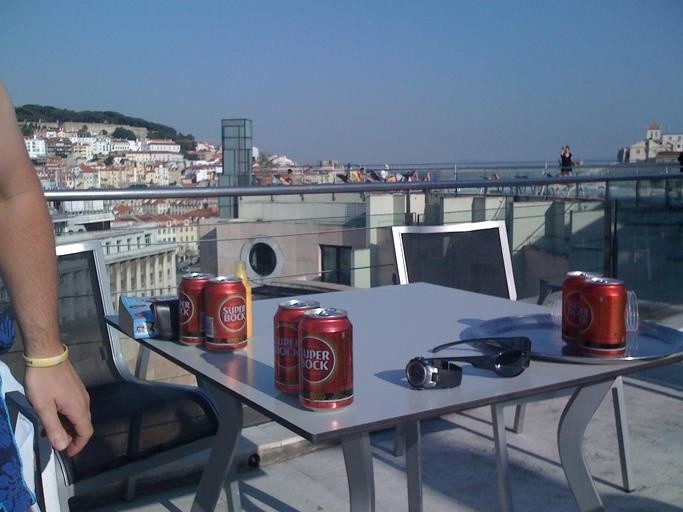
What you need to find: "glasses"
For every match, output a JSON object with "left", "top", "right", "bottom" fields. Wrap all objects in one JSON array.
[{"left": 425, "top": 335, "right": 533, "bottom": 376}]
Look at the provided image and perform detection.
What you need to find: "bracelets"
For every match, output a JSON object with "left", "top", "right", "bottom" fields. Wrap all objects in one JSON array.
[{"left": 22, "top": 344, "right": 69, "bottom": 367}]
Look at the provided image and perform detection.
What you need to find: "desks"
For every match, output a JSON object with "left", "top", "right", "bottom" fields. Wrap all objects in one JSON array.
[{"left": 103, "top": 283, "right": 683, "bottom": 512}]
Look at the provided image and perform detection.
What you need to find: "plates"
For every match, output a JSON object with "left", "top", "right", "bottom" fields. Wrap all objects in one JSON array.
[{"left": 459, "top": 312, "right": 683, "bottom": 363}]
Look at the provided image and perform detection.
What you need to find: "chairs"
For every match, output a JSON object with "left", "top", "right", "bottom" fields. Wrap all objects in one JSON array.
[
  {"left": 644, "top": 170, "right": 672, "bottom": 200},
  {"left": 1, "top": 239, "right": 265, "bottom": 512},
  {"left": 392, "top": 220, "right": 635, "bottom": 512},
  {"left": 479, "top": 173, "right": 606, "bottom": 200},
  {"left": 337, "top": 173, "right": 351, "bottom": 181},
  {"left": 132, "top": 315, "right": 142, "bottom": 316},
  {"left": 274, "top": 175, "right": 290, "bottom": 184}
]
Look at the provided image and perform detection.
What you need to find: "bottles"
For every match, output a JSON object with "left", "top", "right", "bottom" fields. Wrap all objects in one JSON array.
[{"left": 234, "top": 259, "right": 252, "bottom": 339}]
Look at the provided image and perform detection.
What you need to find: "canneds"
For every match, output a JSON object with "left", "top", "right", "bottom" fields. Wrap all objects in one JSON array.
[
  {"left": 562, "top": 271, "right": 603, "bottom": 346},
  {"left": 204, "top": 276, "right": 247, "bottom": 352},
  {"left": 274, "top": 300, "right": 321, "bottom": 395},
  {"left": 179, "top": 275, "right": 208, "bottom": 346},
  {"left": 579, "top": 276, "right": 627, "bottom": 358},
  {"left": 299, "top": 308, "right": 352, "bottom": 411}
]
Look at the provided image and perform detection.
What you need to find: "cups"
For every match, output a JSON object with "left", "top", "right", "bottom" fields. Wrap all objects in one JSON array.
[{"left": 541, "top": 290, "right": 638, "bottom": 333}]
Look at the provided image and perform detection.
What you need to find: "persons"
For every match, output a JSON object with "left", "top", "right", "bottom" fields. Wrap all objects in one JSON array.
[
  {"left": 561, "top": 144, "right": 573, "bottom": 177},
  {"left": 379, "top": 164, "right": 389, "bottom": 179},
  {"left": 0, "top": 80, "right": 94, "bottom": 512}
]
